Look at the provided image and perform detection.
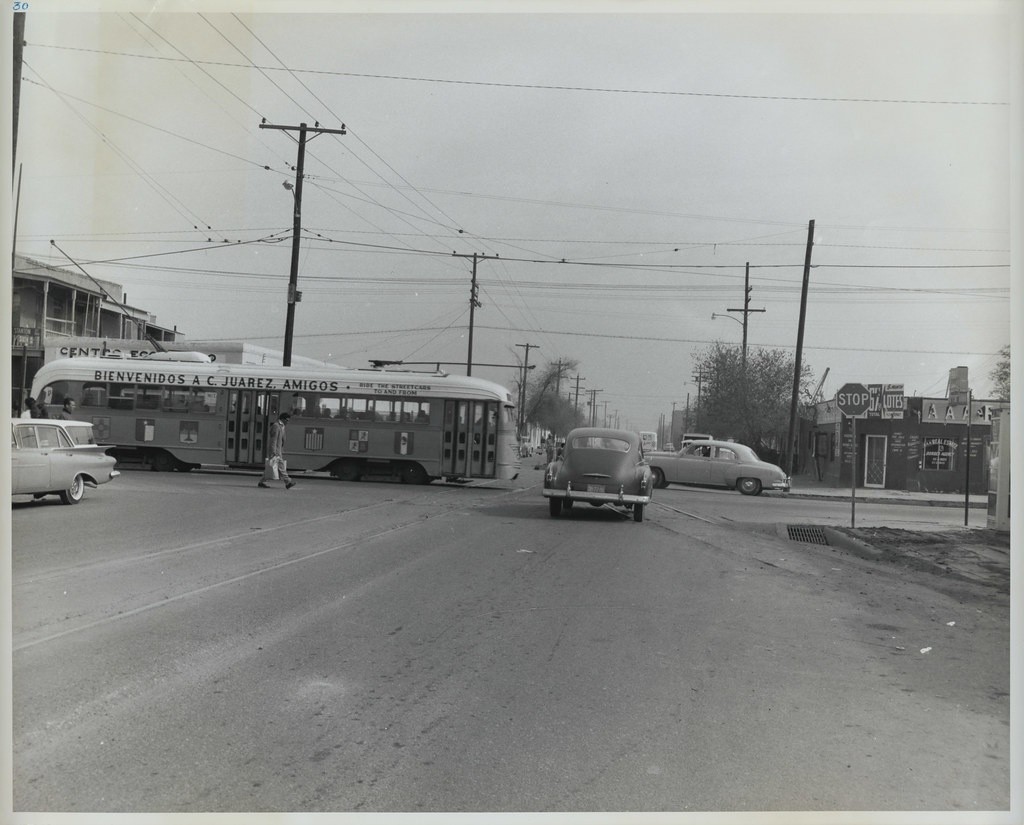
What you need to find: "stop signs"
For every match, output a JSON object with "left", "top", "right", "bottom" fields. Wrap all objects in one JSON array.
[{"left": 833, "top": 382, "right": 870, "bottom": 420}]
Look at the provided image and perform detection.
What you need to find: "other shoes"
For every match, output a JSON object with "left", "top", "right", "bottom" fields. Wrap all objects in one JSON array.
[
  {"left": 258, "top": 482, "right": 271, "bottom": 488},
  {"left": 286, "top": 482, "right": 296, "bottom": 490}
]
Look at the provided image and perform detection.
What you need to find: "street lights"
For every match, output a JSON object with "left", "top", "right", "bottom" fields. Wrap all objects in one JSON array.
[
  {"left": 257, "top": 116, "right": 348, "bottom": 365},
  {"left": 709, "top": 261, "right": 753, "bottom": 439}
]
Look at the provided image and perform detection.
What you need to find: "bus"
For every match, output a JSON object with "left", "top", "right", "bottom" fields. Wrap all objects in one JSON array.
[
  {"left": 639, "top": 431, "right": 657, "bottom": 452},
  {"left": 26, "top": 348, "right": 523, "bottom": 491},
  {"left": 681, "top": 432, "right": 714, "bottom": 453}
]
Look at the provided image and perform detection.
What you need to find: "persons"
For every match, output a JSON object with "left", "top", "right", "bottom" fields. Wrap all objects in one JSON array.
[
  {"left": 24, "top": 397, "right": 43, "bottom": 419},
  {"left": 59, "top": 398, "right": 75, "bottom": 421},
  {"left": 198, "top": 405, "right": 498, "bottom": 426},
  {"left": 258, "top": 413, "right": 296, "bottom": 491},
  {"left": 700, "top": 446, "right": 711, "bottom": 458}
]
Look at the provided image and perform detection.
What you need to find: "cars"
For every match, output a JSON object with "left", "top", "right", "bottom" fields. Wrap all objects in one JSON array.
[
  {"left": 641, "top": 439, "right": 792, "bottom": 499},
  {"left": 543, "top": 427, "right": 655, "bottom": 523},
  {"left": 519, "top": 437, "right": 533, "bottom": 457},
  {"left": 10, "top": 419, "right": 121, "bottom": 509},
  {"left": 662, "top": 441, "right": 676, "bottom": 452},
  {"left": 545, "top": 441, "right": 564, "bottom": 461}
]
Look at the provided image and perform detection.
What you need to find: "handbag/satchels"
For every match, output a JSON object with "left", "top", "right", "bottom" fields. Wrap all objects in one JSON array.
[{"left": 265, "top": 458, "right": 279, "bottom": 481}]
